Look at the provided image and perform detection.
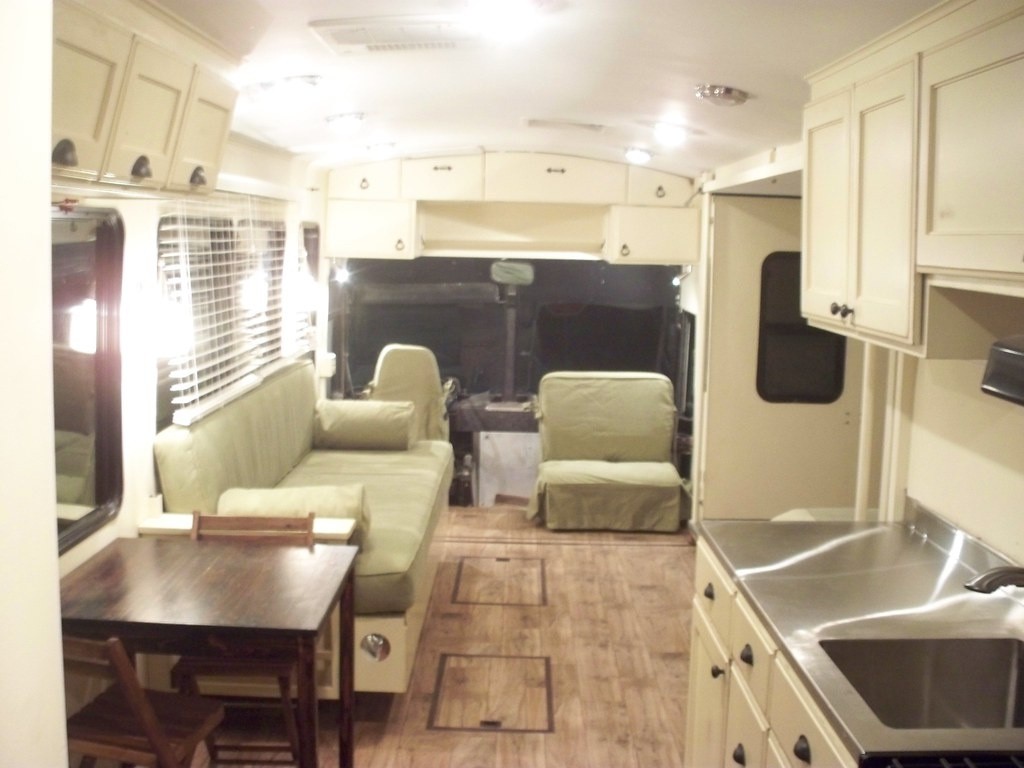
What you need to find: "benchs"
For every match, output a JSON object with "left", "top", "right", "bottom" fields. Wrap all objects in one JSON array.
[
  {"left": 527, "top": 371, "right": 692, "bottom": 533},
  {"left": 136, "top": 359, "right": 456, "bottom": 698}
]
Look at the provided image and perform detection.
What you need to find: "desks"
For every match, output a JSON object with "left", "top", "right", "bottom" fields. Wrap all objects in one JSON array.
[{"left": 60, "top": 537, "right": 359, "bottom": 768}]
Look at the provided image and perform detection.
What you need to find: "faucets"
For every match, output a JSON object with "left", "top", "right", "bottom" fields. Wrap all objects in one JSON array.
[{"left": 964, "top": 566, "right": 1024, "bottom": 594}]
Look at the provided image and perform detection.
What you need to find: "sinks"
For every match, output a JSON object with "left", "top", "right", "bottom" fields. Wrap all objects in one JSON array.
[{"left": 818, "top": 637, "right": 1024, "bottom": 730}]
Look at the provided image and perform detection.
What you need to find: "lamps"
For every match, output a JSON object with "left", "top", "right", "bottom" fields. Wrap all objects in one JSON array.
[{"left": 695, "top": 83, "right": 748, "bottom": 107}]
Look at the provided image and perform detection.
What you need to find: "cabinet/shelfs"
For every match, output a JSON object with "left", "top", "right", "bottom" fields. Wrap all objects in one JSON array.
[
  {"left": 798, "top": 50, "right": 921, "bottom": 347},
  {"left": 682, "top": 540, "right": 858, "bottom": 768}
]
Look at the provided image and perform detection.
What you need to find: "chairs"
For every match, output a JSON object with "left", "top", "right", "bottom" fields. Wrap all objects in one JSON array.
[
  {"left": 167, "top": 506, "right": 314, "bottom": 761},
  {"left": 61, "top": 633, "right": 225, "bottom": 768},
  {"left": 363, "top": 343, "right": 451, "bottom": 442}
]
